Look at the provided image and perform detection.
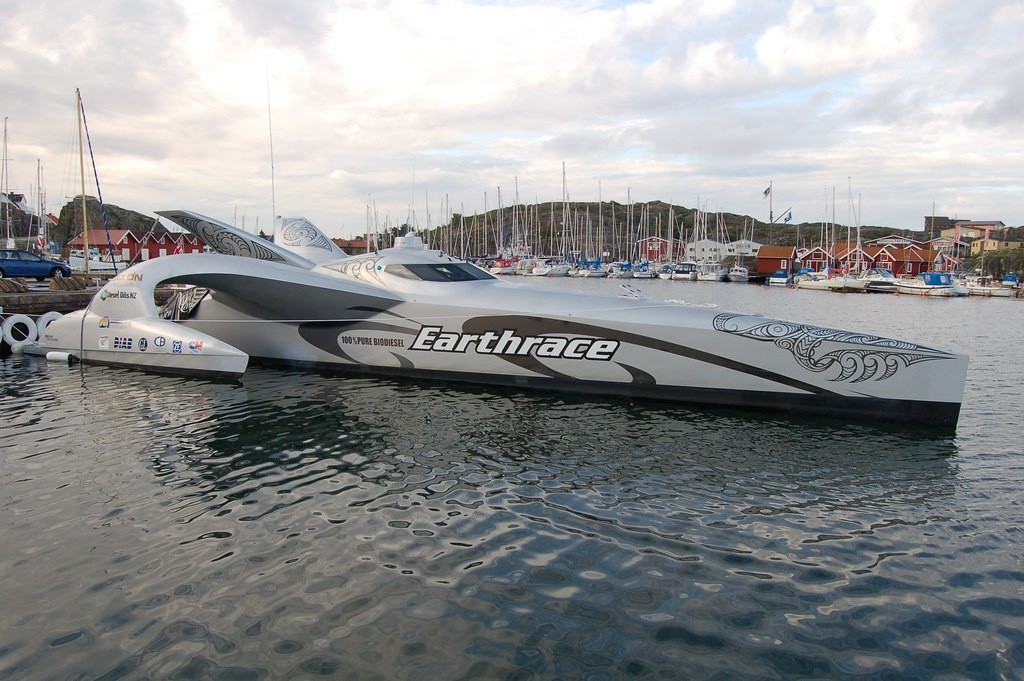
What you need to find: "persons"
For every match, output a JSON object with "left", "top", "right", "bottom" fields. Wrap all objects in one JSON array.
[{"left": 36, "top": 228, "right": 46, "bottom": 253}]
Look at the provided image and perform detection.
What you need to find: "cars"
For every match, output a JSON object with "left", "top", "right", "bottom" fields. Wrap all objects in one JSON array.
[{"left": 0, "top": 249, "right": 72, "bottom": 280}]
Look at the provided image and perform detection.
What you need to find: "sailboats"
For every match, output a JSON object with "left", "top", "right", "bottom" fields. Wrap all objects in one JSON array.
[{"left": 432, "top": 161, "right": 1024, "bottom": 298}]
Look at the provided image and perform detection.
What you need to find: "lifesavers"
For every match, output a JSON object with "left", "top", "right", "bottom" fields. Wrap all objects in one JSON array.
[
  {"left": 3, "top": 315, "right": 37, "bottom": 347},
  {"left": 36, "top": 311, "right": 65, "bottom": 340}
]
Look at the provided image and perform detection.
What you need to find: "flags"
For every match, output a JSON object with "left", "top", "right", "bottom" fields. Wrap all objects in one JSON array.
[
  {"left": 762, "top": 186, "right": 771, "bottom": 199},
  {"left": 784, "top": 211, "right": 792, "bottom": 224}
]
